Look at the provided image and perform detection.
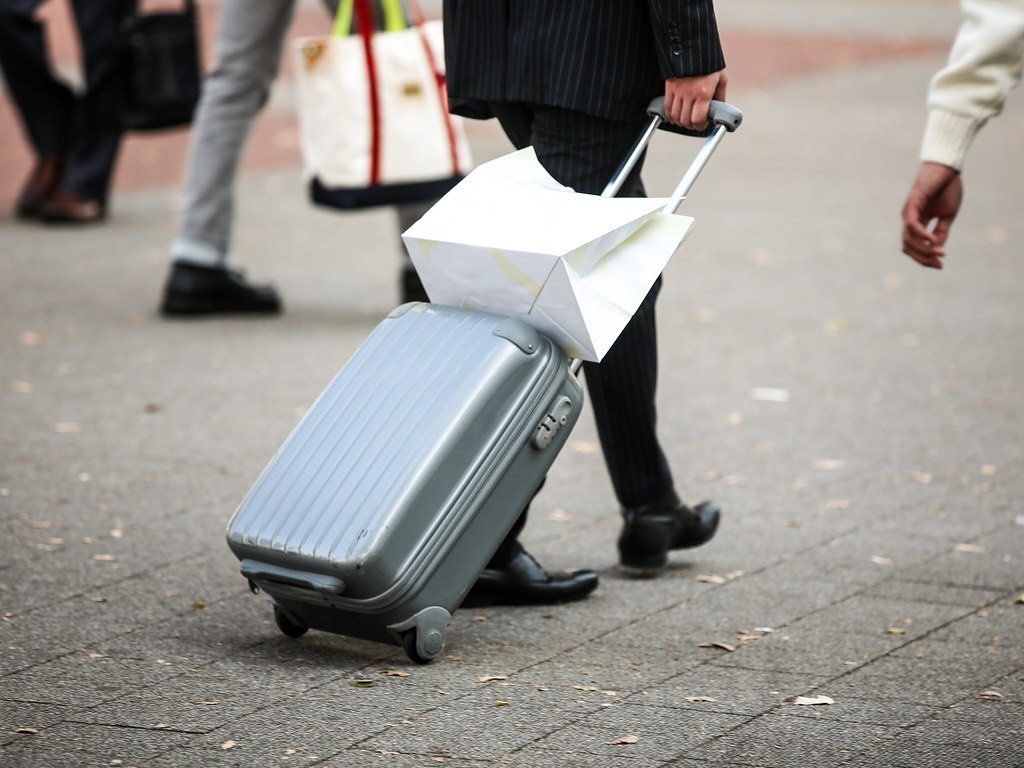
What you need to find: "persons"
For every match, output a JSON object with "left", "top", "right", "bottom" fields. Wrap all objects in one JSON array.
[
  {"left": 901, "top": 0, "right": 1024, "bottom": 270},
  {"left": 441, "top": 0, "right": 727, "bottom": 607},
  {"left": 0, "top": 0, "right": 186, "bottom": 226},
  {"left": 156, "top": 1, "right": 441, "bottom": 318}
]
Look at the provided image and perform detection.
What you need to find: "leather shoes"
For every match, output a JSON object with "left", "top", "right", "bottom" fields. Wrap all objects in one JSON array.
[
  {"left": 460, "top": 552, "right": 598, "bottom": 606},
  {"left": 616, "top": 500, "right": 721, "bottom": 575}
]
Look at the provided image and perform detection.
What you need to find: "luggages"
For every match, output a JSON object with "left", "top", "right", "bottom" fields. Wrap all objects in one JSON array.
[{"left": 224, "top": 94, "right": 742, "bottom": 664}]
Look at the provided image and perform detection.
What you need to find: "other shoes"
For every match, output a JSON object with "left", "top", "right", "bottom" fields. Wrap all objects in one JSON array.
[
  {"left": 16, "top": 151, "right": 116, "bottom": 225},
  {"left": 159, "top": 259, "right": 281, "bottom": 320}
]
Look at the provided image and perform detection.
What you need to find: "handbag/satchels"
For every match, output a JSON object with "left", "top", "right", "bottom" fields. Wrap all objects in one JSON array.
[
  {"left": 114, "top": 0, "right": 202, "bottom": 131},
  {"left": 296, "top": 0, "right": 473, "bottom": 213},
  {"left": 401, "top": 147, "right": 696, "bottom": 364}
]
